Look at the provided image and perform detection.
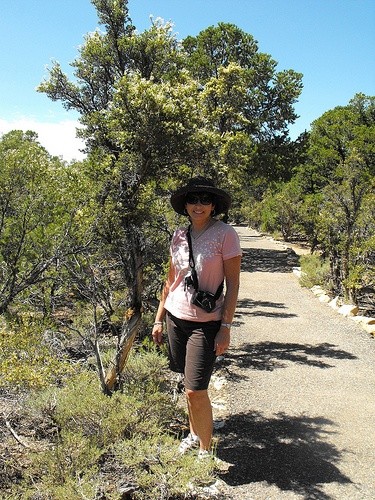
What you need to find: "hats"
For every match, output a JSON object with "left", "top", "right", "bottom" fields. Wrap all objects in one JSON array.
[{"left": 170, "top": 175, "right": 231, "bottom": 218}]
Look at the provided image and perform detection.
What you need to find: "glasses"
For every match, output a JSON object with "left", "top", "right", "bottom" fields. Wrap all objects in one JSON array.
[{"left": 186, "top": 196, "right": 213, "bottom": 206}]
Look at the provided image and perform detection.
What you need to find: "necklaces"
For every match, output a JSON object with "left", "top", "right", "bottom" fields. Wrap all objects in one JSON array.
[{"left": 195, "top": 219, "right": 213, "bottom": 239}]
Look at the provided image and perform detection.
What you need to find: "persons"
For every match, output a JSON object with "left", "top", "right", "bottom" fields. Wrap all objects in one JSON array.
[{"left": 153, "top": 175, "right": 242, "bottom": 461}]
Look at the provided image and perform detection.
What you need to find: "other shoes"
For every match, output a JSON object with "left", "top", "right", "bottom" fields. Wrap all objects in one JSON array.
[
  {"left": 178, "top": 439, "right": 200, "bottom": 457},
  {"left": 193, "top": 450, "right": 215, "bottom": 465}
]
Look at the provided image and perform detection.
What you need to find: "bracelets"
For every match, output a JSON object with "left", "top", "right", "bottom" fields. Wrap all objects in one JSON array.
[
  {"left": 153, "top": 322, "right": 162, "bottom": 325},
  {"left": 221, "top": 323, "right": 231, "bottom": 329}
]
